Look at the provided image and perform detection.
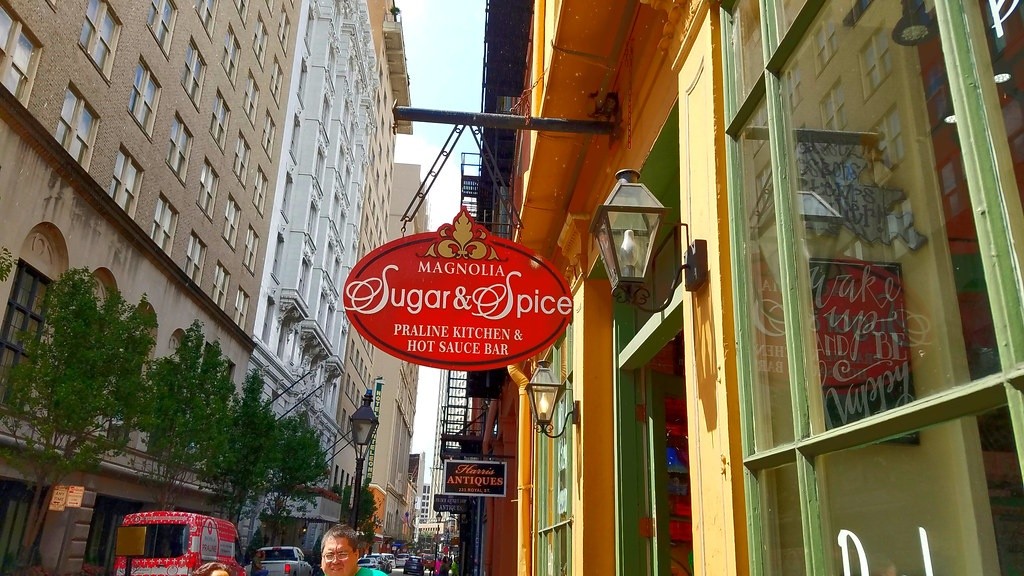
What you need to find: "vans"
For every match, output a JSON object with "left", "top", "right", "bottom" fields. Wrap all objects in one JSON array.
[{"left": 114, "top": 511, "right": 250, "bottom": 576}]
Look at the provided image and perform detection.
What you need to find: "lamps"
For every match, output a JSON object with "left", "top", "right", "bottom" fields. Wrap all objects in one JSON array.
[
  {"left": 302, "top": 522, "right": 308, "bottom": 533},
  {"left": 890, "top": 0, "right": 938, "bottom": 46},
  {"left": 587, "top": 168, "right": 707, "bottom": 312},
  {"left": 524, "top": 360, "right": 580, "bottom": 438}
]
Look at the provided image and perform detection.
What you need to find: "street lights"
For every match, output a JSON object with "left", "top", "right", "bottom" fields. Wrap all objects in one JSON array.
[
  {"left": 348, "top": 389, "right": 380, "bottom": 531},
  {"left": 435, "top": 512, "right": 442, "bottom": 559}
]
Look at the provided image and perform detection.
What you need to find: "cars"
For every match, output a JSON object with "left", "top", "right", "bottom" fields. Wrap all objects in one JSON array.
[
  {"left": 357, "top": 552, "right": 435, "bottom": 576},
  {"left": 403, "top": 556, "right": 424, "bottom": 576}
]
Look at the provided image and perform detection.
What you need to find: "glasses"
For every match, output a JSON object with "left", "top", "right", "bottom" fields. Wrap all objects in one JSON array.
[{"left": 322, "top": 549, "right": 354, "bottom": 562}]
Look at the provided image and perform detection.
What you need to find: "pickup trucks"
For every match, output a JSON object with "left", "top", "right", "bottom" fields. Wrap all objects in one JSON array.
[{"left": 257, "top": 546, "right": 314, "bottom": 576}]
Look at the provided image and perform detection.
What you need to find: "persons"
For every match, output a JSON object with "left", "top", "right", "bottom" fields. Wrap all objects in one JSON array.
[
  {"left": 250, "top": 551, "right": 265, "bottom": 576},
  {"left": 318, "top": 525, "right": 388, "bottom": 576},
  {"left": 195, "top": 563, "right": 237, "bottom": 576},
  {"left": 428, "top": 555, "right": 460, "bottom": 576}
]
